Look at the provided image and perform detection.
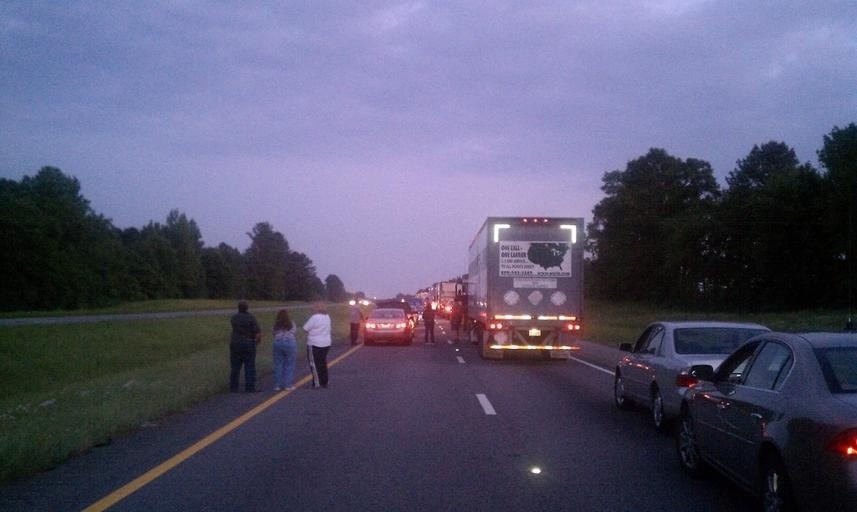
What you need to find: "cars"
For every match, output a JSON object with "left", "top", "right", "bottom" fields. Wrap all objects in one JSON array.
[
  {"left": 678, "top": 331, "right": 856, "bottom": 509},
  {"left": 363, "top": 294, "right": 425, "bottom": 346},
  {"left": 612, "top": 319, "right": 773, "bottom": 433}
]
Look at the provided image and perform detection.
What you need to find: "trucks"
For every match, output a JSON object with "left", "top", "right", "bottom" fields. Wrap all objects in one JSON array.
[{"left": 417, "top": 215, "right": 586, "bottom": 362}]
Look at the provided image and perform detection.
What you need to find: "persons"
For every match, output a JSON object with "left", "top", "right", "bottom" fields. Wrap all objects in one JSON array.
[
  {"left": 446, "top": 298, "right": 463, "bottom": 347},
  {"left": 347, "top": 297, "right": 367, "bottom": 347},
  {"left": 299, "top": 300, "right": 332, "bottom": 389},
  {"left": 422, "top": 301, "right": 436, "bottom": 345},
  {"left": 269, "top": 309, "right": 296, "bottom": 392},
  {"left": 227, "top": 299, "right": 264, "bottom": 394}
]
